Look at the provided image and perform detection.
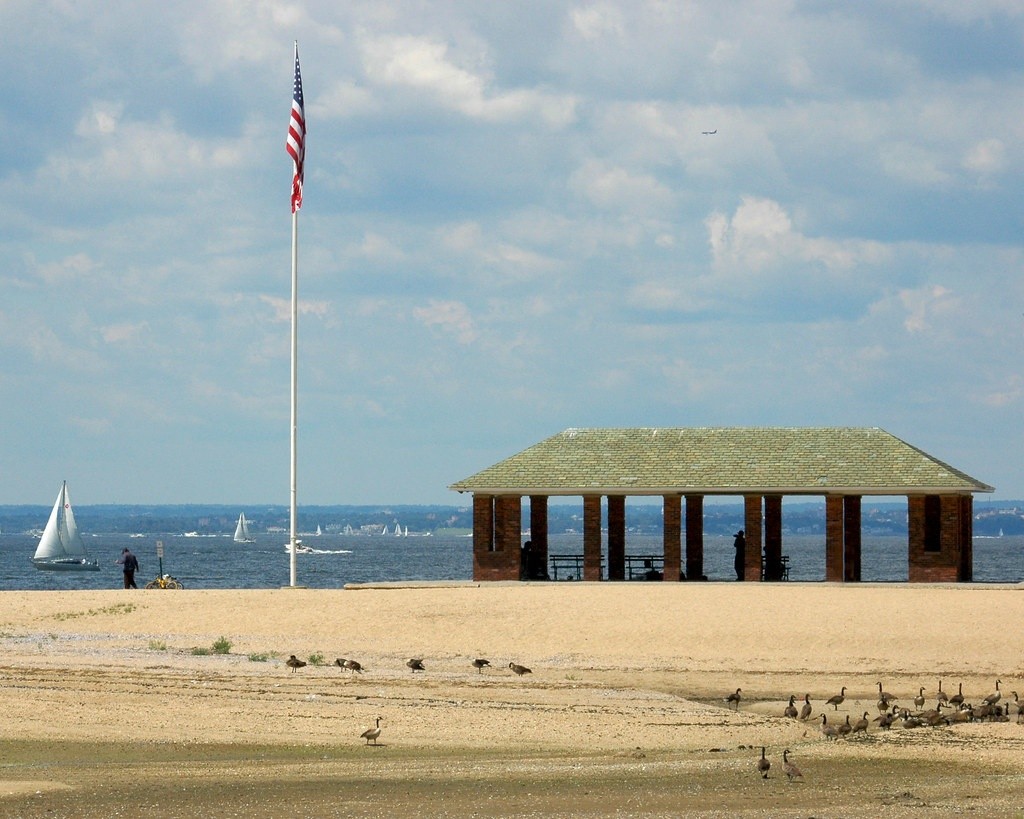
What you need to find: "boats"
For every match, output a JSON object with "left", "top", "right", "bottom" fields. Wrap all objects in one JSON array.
[
  {"left": 184, "top": 532, "right": 199, "bottom": 537},
  {"left": 129, "top": 533, "right": 147, "bottom": 538},
  {"left": 285, "top": 539, "right": 313, "bottom": 553}
]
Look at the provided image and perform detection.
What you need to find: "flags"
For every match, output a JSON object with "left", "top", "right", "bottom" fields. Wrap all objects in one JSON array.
[{"left": 284, "top": 50, "right": 307, "bottom": 214}]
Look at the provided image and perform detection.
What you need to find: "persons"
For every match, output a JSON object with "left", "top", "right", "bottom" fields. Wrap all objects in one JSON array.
[
  {"left": 733, "top": 530, "right": 745, "bottom": 581},
  {"left": 521, "top": 541, "right": 532, "bottom": 580},
  {"left": 114, "top": 548, "right": 141, "bottom": 590}
]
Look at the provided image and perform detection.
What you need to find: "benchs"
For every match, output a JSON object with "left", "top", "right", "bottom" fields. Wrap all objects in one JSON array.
[
  {"left": 625, "top": 554, "right": 664, "bottom": 581},
  {"left": 549, "top": 555, "right": 606, "bottom": 579},
  {"left": 763, "top": 556, "right": 790, "bottom": 581}
]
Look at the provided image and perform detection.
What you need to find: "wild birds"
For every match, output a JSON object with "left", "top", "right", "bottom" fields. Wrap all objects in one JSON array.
[
  {"left": 509, "top": 661, "right": 532, "bottom": 677},
  {"left": 781, "top": 750, "right": 802, "bottom": 783},
  {"left": 406, "top": 658, "right": 425, "bottom": 673},
  {"left": 872, "top": 702, "right": 1011, "bottom": 728},
  {"left": 818, "top": 713, "right": 838, "bottom": 741},
  {"left": 784, "top": 695, "right": 798, "bottom": 719},
  {"left": 838, "top": 715, "right": 852, "bottom": 739},
  {"left": 914, "top": 687, "right": 927, "bottom": 711},
  {"left": 360, "top": 717, "right": 383, "bottom": 746},
  {"left": 286, "top": 655, "right": 307, "bottom": 673},
  {"left": 723, "top": 688, "right": 743, "bottom": 712},
  {"left": 875, "top": 682, "right": 898, "bottom": 714},
  {"left": 472, "top": 659, "right": 492, "bottom": 674},
  {"left": 852, "top": 712, "right": 870, "bottom": 734},
  {"left": 758, "top": 747, "right": 771, "bottom": 779},
  {"left": 936, "top": 678, "right": 1024, "bottom": 725},
  {"left": 799, "top": 694, "right": 813, "bottom": 721},
  {"left": 825, "top": 686, "right": 848, "bottom": 711},
  {"left": 336, "top": 658, "right": 364, "bottom": 675}
]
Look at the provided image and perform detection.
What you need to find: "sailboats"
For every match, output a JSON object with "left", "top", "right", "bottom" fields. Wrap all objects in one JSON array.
[
  {"left": 30, "top": 480, "right": 101, "bottom": 572},
  {"left": 315, "top": 524, "right": 322, "bottom": 536},
  {"left": 404, "top": 525, "right": 408, "bottom": 538},
  {"left": 394, "top": 523, "right": 402, "bottom": 536},
  {"left": 381, "top": 525, "right": 388, "bottom": 535},
  {"left": 234, "top": 511, "right": 255, "bottom": 543}
]
